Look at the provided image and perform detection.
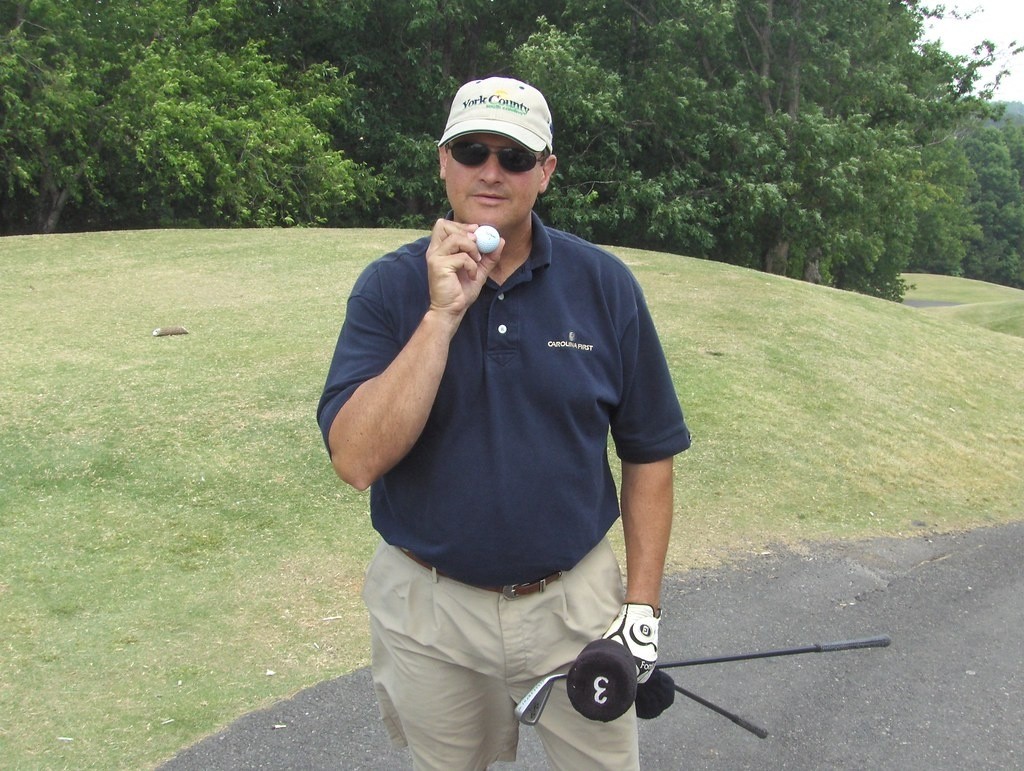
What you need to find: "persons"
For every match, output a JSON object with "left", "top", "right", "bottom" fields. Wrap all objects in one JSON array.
[{"left": 315, "top": 75, "right": 693, "bottom": 771}]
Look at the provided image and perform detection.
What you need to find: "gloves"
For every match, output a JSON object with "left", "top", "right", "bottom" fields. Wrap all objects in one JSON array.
[{"left": 601, "top": 601, "right": 663, "bottom": 683}]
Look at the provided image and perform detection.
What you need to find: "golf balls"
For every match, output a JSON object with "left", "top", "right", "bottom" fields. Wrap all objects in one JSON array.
[{"left": 472, "top": 225, "right": 500, "bottom": 254}]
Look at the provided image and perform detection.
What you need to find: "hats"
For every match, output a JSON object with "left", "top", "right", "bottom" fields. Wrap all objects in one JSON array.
[{"left": 438, "top": 77, "right": 553, "bottom": 154}]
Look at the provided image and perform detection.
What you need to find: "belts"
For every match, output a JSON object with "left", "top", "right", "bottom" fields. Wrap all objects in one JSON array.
[{"left": 399, "top": 547, "right": 563, "bottom": 600}]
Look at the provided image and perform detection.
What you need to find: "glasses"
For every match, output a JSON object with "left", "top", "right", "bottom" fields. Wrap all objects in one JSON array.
[{"left": 447, "top": 140, "right": 545, "bottom": 173}]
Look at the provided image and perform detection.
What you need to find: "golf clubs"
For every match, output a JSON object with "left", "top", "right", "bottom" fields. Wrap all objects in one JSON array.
[
  {"left": 659, "top": 674, "right": 769, "bottom": 740},
  {"left": 514, "top": 637, "right": 892, "bottom": 726}
]
